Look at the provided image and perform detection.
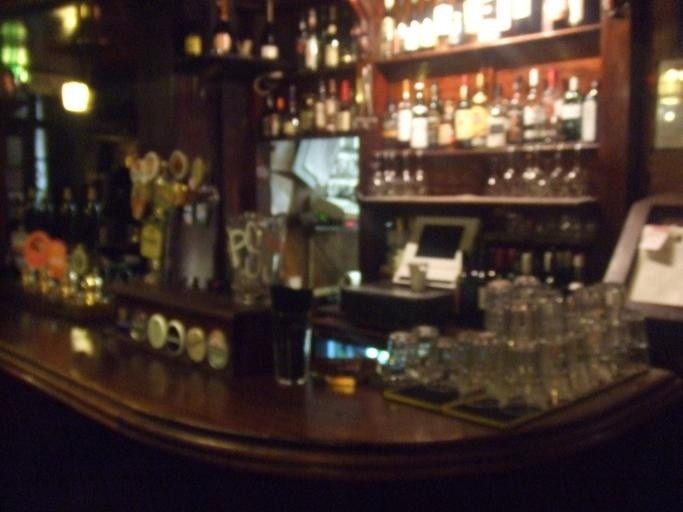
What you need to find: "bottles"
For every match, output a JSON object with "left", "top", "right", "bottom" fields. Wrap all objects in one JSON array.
[
  {"left": 467, "top": 248, "right": 590, "bottom": 325},
  {"left": 10, "top": 179, "right": 109, "bottom": 310},
  {"left": 378, "top": 1, "right": 595, "bottom": 64},
  {"left": 260, "top": 73, "right": 371, "bottom": 135},
  {"left": 376, "top": 63, "right": 595, "bottom": 150},
  {"left": 184, "top": 1, "right": 366, "bottom": 67}
]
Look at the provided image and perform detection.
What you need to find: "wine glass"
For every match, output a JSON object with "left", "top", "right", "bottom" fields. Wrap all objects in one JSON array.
[{"left": 364, "top": 147, "right": 590, "bottom": 198}]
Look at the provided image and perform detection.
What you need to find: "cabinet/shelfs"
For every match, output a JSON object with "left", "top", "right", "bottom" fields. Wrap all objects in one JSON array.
[
  {"left": 255, "top": 58, "right": 354, "bottom": 138},
  {"left": 355, "top": 20, "right": 597, "bottom": 208}
]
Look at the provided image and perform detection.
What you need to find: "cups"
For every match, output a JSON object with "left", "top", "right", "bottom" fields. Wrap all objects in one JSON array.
[
  {"left": 408, "top": 261, "right": 427, "bottom": 292},
  {"left": 224, "top": 211, "right": 284, "bottom": 306},
  {"left": 273, "top": 322, "right": 310, "bottom": 387},
  {"left": 382, "top": 282, "right": 655, "bottom": 410}
]
